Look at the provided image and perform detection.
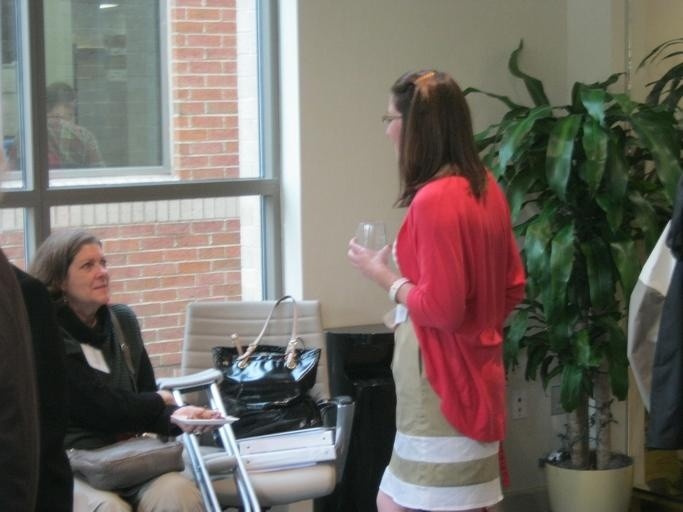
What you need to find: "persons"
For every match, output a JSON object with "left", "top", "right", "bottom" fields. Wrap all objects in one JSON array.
[
  {"left": 345, "top": 68, "right": 529, "bottom": 511},
  {"left": 7, "top": 82, "right": 107, "bottom": 170},
  {"left": 25, "top": 226, "right": 224, "bottom": 512},
  {"left": 0, "top": 251, "right": 76, "bottom": 512}
]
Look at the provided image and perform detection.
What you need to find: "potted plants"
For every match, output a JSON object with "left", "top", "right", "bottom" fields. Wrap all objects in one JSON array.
[{"left": 460, "top": 36, "right": 683, "bottom": 512}]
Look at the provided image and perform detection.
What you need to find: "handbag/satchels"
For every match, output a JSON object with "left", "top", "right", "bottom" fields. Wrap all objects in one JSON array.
[
  {"left": 65, "top": 431, "right": 185, "bottom": 491},
  {"left": 210, "top": 295, "right": 323, "bottom": 449}
]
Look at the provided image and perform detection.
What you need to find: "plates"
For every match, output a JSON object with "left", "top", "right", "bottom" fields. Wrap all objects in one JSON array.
[{"left": 168, "top": 413, "right": 240, "bottom": 425}]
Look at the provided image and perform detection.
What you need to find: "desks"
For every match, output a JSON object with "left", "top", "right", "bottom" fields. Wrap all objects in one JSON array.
[{"left": 313, "top": 325, "right": 396, "bottom": 512}]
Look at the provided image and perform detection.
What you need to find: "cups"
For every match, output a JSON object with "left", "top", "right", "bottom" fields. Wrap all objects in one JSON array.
[{"left": 353, "top": 221, "right": 388, "bottom": 270}]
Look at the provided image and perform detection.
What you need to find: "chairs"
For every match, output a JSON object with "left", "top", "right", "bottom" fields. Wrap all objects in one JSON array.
[{"left": 175, "top": 297, "right": 355, "bottom": 512}]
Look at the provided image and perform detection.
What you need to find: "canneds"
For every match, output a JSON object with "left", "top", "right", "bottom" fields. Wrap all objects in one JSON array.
[{"left": 0, "top": 136, "right": 17, "bottom": 170}]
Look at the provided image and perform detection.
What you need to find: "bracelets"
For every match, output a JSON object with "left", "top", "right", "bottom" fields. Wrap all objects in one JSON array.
[{"left": 388, "top": 275, "right": 411, "bottom": 306}]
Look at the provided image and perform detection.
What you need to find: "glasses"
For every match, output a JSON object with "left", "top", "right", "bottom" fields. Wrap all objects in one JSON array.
[{"left": 382, "top": 115, "right": 401, "bottom": 128}]
